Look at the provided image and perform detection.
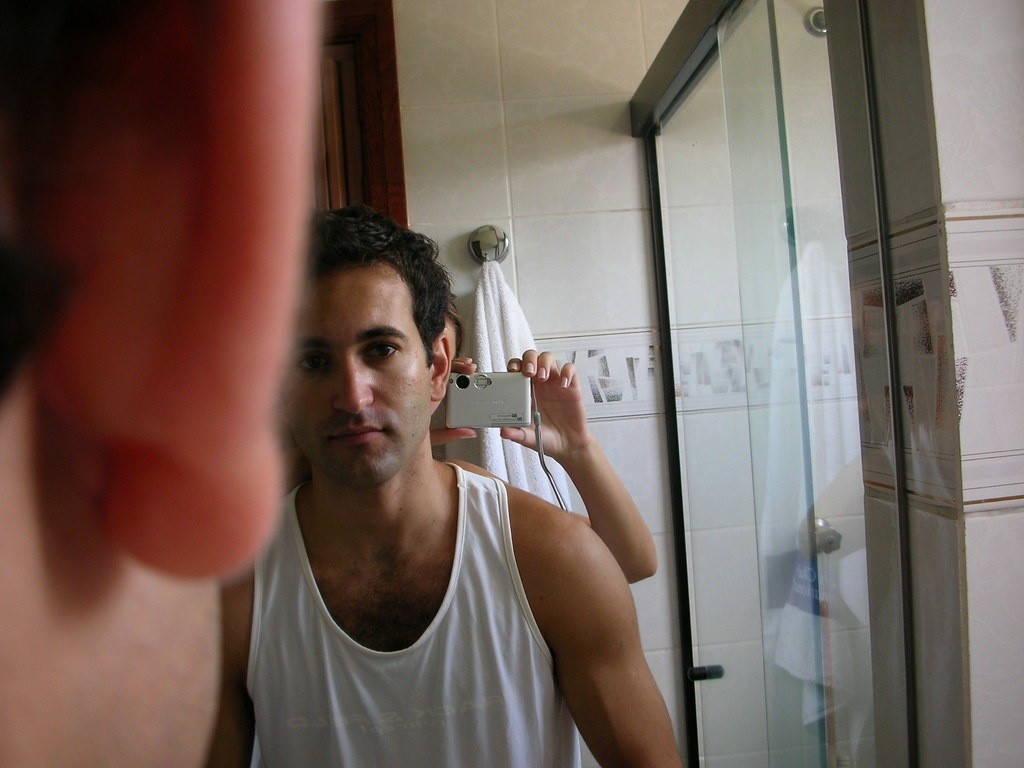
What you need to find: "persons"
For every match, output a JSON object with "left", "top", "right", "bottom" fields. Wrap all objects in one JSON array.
[
  {"left": 203, "top": 209, "right": 688, "bottom": 768},
  {"left": 0, "top": 0, "right": 318, "bottom": 768}
]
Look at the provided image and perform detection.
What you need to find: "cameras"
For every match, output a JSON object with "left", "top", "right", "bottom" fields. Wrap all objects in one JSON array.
[{"left": 447, "top": 371, "right": 532, "bottom": 428}]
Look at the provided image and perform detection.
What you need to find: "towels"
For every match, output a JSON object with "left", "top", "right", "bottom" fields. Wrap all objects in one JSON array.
[
  {"left": 765, "top": 243, "right": 876, "bottom": 768},
  {"left": 444, "top": 257, "right": 572, "bottom": 515}
]
